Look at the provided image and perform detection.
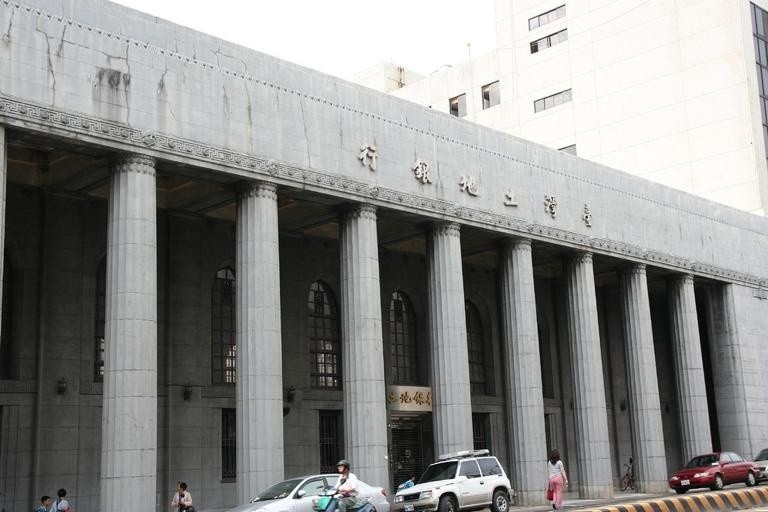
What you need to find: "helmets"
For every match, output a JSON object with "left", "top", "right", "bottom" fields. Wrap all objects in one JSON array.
[{"left": 337, "top": 460, "right": 351, "bottom": 470}]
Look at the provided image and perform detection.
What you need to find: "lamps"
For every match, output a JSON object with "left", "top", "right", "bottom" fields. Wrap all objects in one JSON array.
[{"left": 57, "top": 376, "right": 68, "bottom": 394}]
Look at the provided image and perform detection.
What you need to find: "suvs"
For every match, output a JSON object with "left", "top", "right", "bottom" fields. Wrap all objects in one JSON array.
[{"left": 391, "top": 447, "right": 517, "bottom": 511}]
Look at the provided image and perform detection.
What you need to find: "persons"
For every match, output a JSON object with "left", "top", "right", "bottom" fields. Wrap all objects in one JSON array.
[
  {"left": 35, "top": 495, "right": 53, "bottom": 512},
  {"left": 545, "top": 449, "right": 568, "bottom": 509},
  {"left": 398, "top": 467, "right": 415, "bottom": 489},
  {"left": 169, "top": 480, "right": 192, "bottom": 512},
  {"left": 49, "top": 488, "right": 70, "bottom": 512},
  {"left": 329, "top": 457, "right": 358, "bottom": 512}
]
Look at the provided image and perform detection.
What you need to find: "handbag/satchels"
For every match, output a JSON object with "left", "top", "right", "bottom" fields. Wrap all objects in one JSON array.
[{"left": 547, "top": 483, "right": 553, "bottom": 500}]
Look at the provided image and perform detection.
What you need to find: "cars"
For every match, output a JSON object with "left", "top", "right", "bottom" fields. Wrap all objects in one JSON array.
[
  {"left": 223, "top": 471, "right": 393, "bottom": 511},
  {"left": 747, "top": 445, "right": 767, "bottom": 485},
  {"left": 667, "top": 448, "right": 759, "bottom": 495}
]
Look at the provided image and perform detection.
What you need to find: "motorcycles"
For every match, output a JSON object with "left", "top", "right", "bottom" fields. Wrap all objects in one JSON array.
[{"left": 314, "top": 485, "right": 377, "bottom": 512}]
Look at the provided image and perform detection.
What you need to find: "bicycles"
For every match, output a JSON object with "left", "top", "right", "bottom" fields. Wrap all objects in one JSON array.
[{"left": 618, "top": 456, "right": 635, "bottom": 492}]
